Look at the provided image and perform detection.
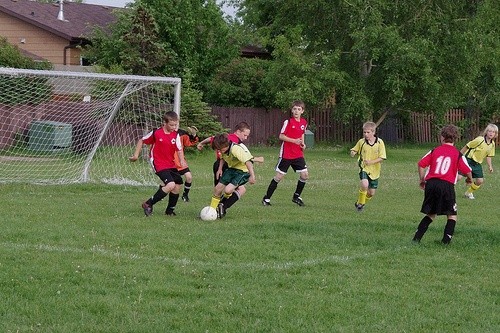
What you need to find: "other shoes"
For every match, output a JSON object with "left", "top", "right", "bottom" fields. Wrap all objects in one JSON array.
[{"left": 182, "top": 196, "right": 190, "bottom": 202}]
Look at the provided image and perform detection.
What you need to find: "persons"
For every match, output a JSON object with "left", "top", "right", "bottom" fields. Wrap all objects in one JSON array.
[
  {"left": 350, "top": 121, "right": 386, "bottom": 211},
  {"left": 128, "top": 111, "right": 187, "bottom": 216},
  {"left": 412, "top": 126, "right": 473, "bottom": 246},
  {"left": 197, "top": 121, "right": 264, "bottom": 220},
  {"left": 261, "top": 100, "right": 308, "bottom": 207},
  {"left": 209, "top": 132, "right": 255, "bottom": 210},
  {"left": 176, "top": 125, "right": 200, "bottom": 204},
  {"left": 458, "top": 123, "right": 498, "bottom": 199}
]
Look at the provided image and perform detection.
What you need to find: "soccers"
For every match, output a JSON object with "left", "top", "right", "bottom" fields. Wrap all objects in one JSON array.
[{"left": 200, "top": 206, "right": 217, "bottom": 221}]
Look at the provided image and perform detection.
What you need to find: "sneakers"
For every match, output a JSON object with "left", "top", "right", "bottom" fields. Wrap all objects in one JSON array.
[
  {"left": 355, "top": 202, "right": 364, "bottom": 213},
  {"left": 262, "top": 195, "right": 271, "bottom": 206},
  {"left": 165, "top": 210, "right": 177, "bottom": 217},
  {"left": 464, "top": 191, "right": 475, "bottom": 200},
  {"left": 292, "top": 194, "right": 305, "bottom": 207},
  {"left": 142, "top": 202, "right": 153, "bottom": 217},
  {"left": 217, "top": 204, "right": 225, "bottom": 219}
]
